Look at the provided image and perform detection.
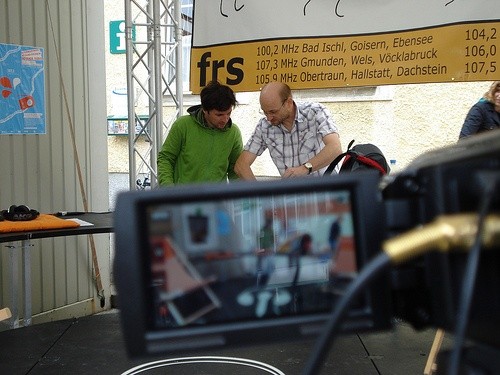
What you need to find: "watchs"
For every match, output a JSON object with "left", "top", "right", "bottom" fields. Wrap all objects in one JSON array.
[{"left": 303, "top": 162, "right": 312, "bottom": 175}]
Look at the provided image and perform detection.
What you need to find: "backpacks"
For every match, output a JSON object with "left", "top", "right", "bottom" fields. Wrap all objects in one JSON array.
[{"left": 322, "top": 144, "right": 391, "bottom": 177}]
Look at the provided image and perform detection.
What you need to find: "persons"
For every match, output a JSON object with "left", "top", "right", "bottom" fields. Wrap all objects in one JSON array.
[
  {"left": 251, "top": 212, "right": 345, "bottom": 293},
  {"left": 233, "top": 80, "right": 343, "bottom": 182},
  {"left": 157, "top": 80, "right": 243, "bottom": 187},
  {"left": 457, "top": 81, "right": 500, "bottom": 139}
]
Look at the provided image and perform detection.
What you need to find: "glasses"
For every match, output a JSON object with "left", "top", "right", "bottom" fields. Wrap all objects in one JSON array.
[{"left": 259, "top": 99, "right": 287, "bottom": 116}]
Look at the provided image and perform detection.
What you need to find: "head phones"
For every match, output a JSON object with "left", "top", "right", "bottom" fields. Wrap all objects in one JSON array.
[{"left": 0, "top": 204, "right": 40, "bottom": 221}]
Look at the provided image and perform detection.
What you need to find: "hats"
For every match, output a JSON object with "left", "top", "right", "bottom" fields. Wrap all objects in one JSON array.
[{"left": 3, "top": 205, "right": 32, "bottom": 220}]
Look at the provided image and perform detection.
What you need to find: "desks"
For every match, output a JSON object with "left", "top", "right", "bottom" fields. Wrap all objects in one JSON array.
[{"left": 0, "top": 211, "right": 122, "bottom": 328}]
